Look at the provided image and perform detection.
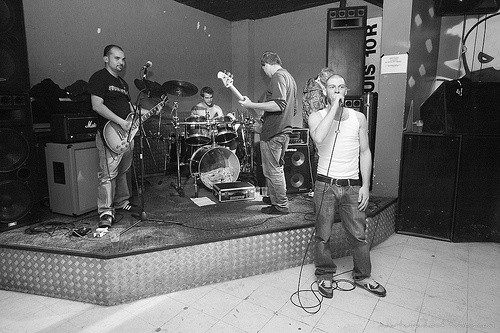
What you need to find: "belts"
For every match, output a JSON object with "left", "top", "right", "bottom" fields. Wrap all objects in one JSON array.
[{"left": 316, "top": 175, "right": 361, "bottom": 187}]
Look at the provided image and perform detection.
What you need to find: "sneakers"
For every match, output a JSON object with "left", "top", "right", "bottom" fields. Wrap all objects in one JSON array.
[
  {"left": 114, "top": 202, "right": 138, "bottom": 212},
  {"left": 354, "top": 276, "right": 387, "bottom": 297},
  {"left": 318, "top": 280, "right": 334, "bottom": 298},
  {"left": 98, "top": 215, "right": 112, "bottom": 228}
]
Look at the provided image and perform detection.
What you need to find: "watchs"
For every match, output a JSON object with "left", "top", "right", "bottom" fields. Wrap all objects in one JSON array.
[{"left": 238, "top": 51, "right": 298, "bottom": 213}]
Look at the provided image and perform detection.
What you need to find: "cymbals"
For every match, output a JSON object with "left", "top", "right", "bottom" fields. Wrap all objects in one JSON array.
[
  {"left": 161, "top": 79, "right": 199, "bottom": 98},
  {"left": 133, "top": 78, "right": 166, "bottom": 98}
]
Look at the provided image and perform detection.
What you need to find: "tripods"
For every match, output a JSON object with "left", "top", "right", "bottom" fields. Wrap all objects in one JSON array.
[{"left": 118, "top": 75, "right": 184, "bottom": 236}]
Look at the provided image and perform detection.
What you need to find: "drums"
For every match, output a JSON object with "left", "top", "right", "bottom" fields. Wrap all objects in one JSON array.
[
  {"left": 211, "top": 115, "right": 239, "bottom": 144},
  {"left": 184, "top": 115, "right": 211, "bottom": 146},
  {"left": 189, "top": 144, "right": 241, "bottom": 190}
]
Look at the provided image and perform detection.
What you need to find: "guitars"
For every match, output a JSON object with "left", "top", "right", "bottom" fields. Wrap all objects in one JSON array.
[
  {"left": 100, "top": 95, "right": 170, "bottom": 155},
  {"left": 216, "top": 69, "right": 265, "bottom": 135}
]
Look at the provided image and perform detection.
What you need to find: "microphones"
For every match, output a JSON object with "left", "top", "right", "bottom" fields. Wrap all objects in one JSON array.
[
  {"left": 339, "top": 100, "right": 343, "bottom": 107},
  {"left": 140, "top": 61, "right": 152, "bottom": 71}
]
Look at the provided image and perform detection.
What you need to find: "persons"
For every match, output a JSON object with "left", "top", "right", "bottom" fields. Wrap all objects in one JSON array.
[
  {"left": 308, "top": 74, "right": 386, "bottom": 298},
  {"left": 303, "top": 68, "right": 334, "bottom": 189},
  {"left": 87, "top": 45, "right": 163, "bottom": 227},
  {"left": 190, "top": 86, "right": 223, "bottom": 122}
]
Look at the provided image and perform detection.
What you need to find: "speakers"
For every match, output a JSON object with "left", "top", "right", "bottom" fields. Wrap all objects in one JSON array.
[
  {"left": 434, "top": 0, "right": 500, "bottom": 17},
  {"left": 0, "top": 0, "right": 46, "bottom": 231},
  {"left": 257, "top": 145, "right": 315, "bottom": 194},
  {"left": 396, "top": 132, "right": 500, "bottom": 244},
  {"left": 419, "top": 74, "right": 500, "bottom": 134},
  {"left": 326, "top": 6, "right": 367, "bottom": 98},
  {"left": 45, "top": 142, "right": 132, "bottom": 216}
]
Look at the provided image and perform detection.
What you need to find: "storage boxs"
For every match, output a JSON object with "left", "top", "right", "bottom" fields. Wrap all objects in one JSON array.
[{"left": 213, "top": 182, "right": 256, "bottom": 203}]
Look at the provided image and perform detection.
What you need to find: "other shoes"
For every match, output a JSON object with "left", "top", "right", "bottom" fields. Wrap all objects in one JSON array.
[
  {"left": 262, "top": 197, "right": 272, "bottom": 205},
  {"left": 261, "top": 205, "right": 289, "bottom": 214}
]
